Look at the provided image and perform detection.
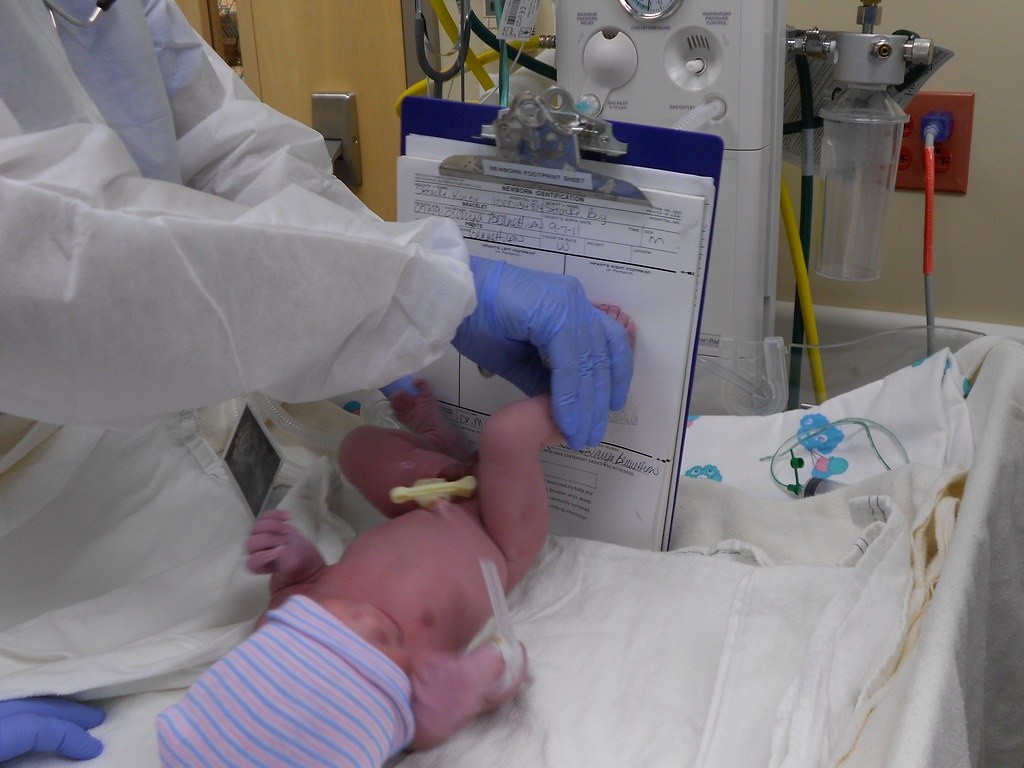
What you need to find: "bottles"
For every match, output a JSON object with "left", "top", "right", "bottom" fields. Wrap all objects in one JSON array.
[{"left": 814, "top": 86, "right": 910, "bottom": 283}]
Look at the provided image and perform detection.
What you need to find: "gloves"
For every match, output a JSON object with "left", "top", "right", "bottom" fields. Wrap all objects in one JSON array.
[
  {"left": 449, "top": 255, "right": 634, "bottom": 452},
  {"left": 0, "top": 696, "right": 108, "bottom": 767},
  {"left": 378, "top": 374, "right": 421, "bottom": 402}
]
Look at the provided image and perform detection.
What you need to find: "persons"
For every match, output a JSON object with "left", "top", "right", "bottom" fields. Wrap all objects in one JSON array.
[
  {"left": 0, "top": 2, "right": 632, "bottom": 702},
  {"left": 159, "top": 301, "right": 639, "bottom": 765}
]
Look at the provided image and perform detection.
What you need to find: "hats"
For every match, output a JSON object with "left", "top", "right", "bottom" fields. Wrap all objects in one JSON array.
[{"left": 155, "top": 591, "right": 415, "bottom": 768}]
